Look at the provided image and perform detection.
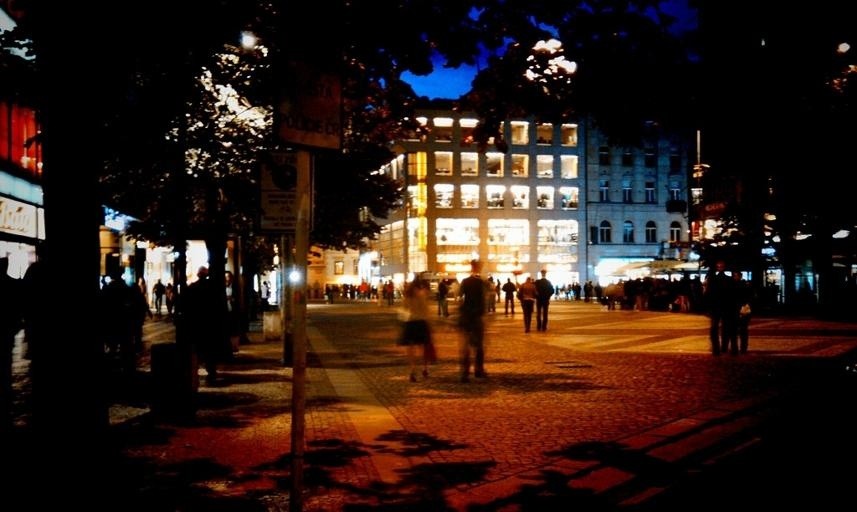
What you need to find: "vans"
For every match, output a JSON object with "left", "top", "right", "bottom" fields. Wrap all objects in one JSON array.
[{"left": 421, "top": 274, "right": 460, "bottom": 297}]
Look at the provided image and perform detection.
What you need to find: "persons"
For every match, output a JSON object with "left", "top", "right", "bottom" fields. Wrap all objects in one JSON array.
[
  {"left": 399, "top": 259, "right": 501, "bottom": 382},
  {"left": 502, "top": 268, "right": 554, "bottom": 331},
  {"left": 1, "top": 242, "right": 269, "bottom": 386},
  {"left": 555, "top": 276, "right": 751, "bottom": 313},
  {"left": 699, "top": 261, "right": 751, "bottom": 354},
  {"left": 308, "top": 278, "right": 394, "bottom": 305}
]
